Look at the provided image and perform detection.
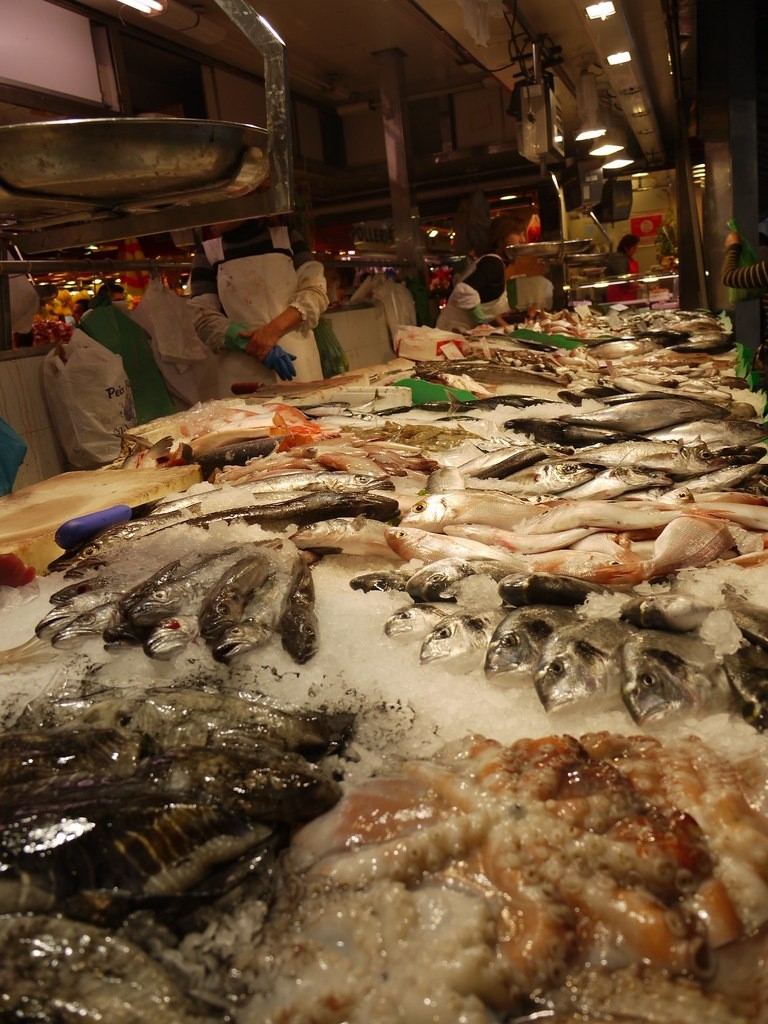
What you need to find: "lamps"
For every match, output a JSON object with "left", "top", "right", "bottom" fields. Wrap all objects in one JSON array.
[{"left": 570, "top": 53, "right": 635, "bottom": 170}]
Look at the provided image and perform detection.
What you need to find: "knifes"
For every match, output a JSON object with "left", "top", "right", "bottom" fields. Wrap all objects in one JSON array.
[
  {"left": 54, "top": 489, "right": 188, "bottom": 549},
  {"left": 231, "top": 381, "right": 320, "bottom": 397}
]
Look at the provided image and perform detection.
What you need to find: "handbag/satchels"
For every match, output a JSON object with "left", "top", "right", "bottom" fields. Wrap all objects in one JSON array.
[
  {"left": 726, "top": 219, "right": 765, "bottom": 305},
  {"left": 41, "top": 329, "right": 136, "bottom": 470},
  {"left": 312, "top": 318, "right": 349, "bottom": 379}
]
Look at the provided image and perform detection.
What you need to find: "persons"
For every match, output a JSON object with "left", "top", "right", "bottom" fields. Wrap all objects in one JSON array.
[
  {"left": 605, "top": 234, "right": 658, "bottom": 302},
  {"left": 6, "top": 241, "right": 125, "bottom": 348},
  {"left": 190, "top": 219, "right": 330, "bottom": 397},
  {"left": 720, "top": 231, "right": 768, "bottom": 386},
  {"left": 343, "top": 214, "right": 527, "bottom": 352}
]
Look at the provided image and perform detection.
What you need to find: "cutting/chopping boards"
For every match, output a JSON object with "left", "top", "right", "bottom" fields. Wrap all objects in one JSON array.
[
  {"left": 221, "top": 375, "right": 369, "bottom": 404},
  {"left": 0, "top": 464, "right": 203, "bottom": 576}
]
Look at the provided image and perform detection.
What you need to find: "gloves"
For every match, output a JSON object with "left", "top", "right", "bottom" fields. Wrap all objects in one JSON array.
[
  {"left": 224, "top": 324, "right": 299, "bottom": 381},
  {"left": 469, "top": 305, "right": 488, "bottom": 324}
]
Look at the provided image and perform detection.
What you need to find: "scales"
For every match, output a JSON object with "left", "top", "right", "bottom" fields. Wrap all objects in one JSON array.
[
  {"left": 0, "top": 0, "right": 297, "bottom": 256},
  {"left": 564, "top": 159, "right": 610, "bottom": 265},
  {"left": 504, "top": 82, "right": 594, "bottom": 265}
]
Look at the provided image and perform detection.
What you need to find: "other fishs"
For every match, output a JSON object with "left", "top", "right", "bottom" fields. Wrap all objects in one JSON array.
[
  {"left": 1, "top": 681, "right": 359, "bottom": 1024},
  {"left": 34, "top": 310, "right": 768, "bottom": 736}
]
